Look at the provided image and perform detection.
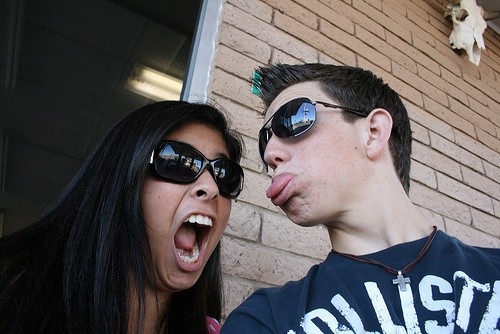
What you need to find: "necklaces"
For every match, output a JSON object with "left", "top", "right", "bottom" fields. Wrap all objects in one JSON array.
[{"left": 329, "top": 224, "right": 438, "bottom": 293}]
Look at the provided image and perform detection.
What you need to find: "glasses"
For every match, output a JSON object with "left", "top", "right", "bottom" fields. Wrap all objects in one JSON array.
[
  {"left": 258, "top": 97, "right": 369, "bottom": 173},
  {"left": 142, "top": 140, "right": 244, "bottom": 199}
]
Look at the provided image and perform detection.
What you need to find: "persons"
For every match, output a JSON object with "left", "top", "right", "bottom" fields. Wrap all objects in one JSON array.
[
  {"left": 0, "top": 99, "right": 244, "bottom": 334},
  {"left": 219, "top": 64, "right": 500, "bottom": 334}
]
